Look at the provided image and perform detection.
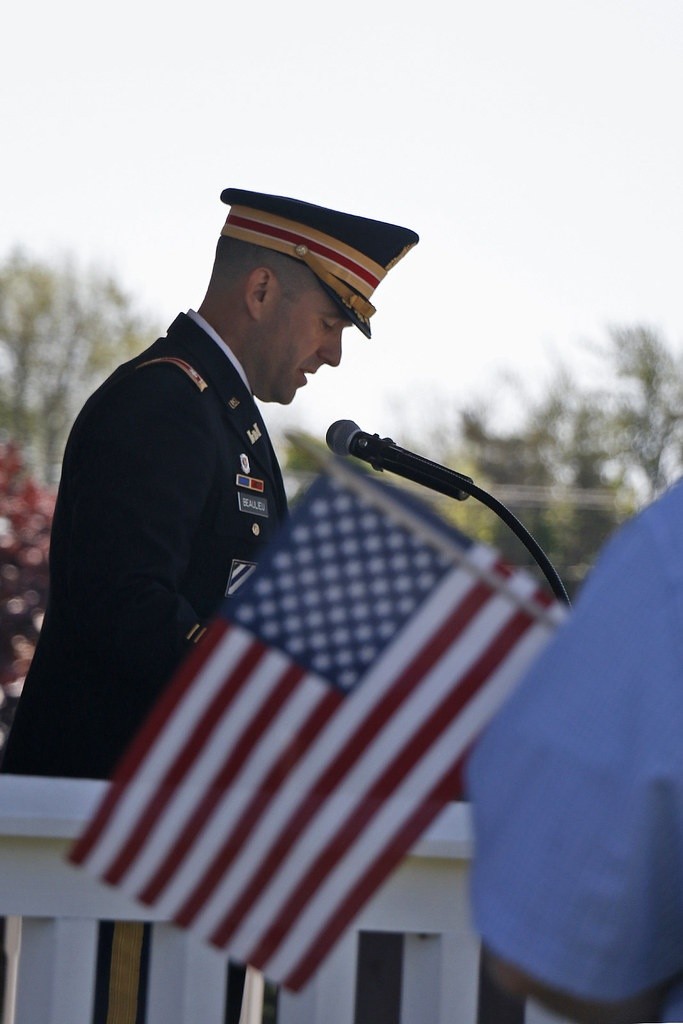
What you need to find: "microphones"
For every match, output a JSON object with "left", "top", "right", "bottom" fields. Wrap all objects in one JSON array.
[{"left": 327, "top": 419, "right": 474, "bottom": 503}]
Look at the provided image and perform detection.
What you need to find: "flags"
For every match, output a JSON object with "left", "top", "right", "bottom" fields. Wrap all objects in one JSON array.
[{"left": 64, "top": 428, "right": 565, "bottom": 996}]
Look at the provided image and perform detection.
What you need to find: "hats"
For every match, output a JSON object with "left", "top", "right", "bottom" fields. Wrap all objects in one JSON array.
[{"left": 218, "top": 189, "right": 418, "bottom": 338}]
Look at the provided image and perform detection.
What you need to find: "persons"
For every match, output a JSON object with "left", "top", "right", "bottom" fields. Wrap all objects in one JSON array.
[
  {"left": 0, "top": 188, "right": 419, "bottom": 1024},
  {"left": 471, "top": 464, "right": 683, "bottom": 1024}
]
